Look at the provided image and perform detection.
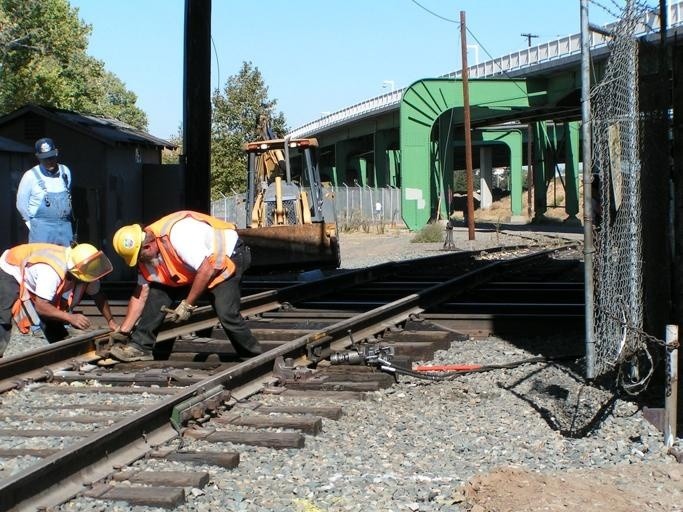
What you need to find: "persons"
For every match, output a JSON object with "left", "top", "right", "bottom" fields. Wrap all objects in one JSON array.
[
  {"left": 108, "top": 209, "right": 264, "bottom": 364},
  {"left": 15, "top": 138, "right": 74, "bottom": 340},
  {"left": 0, "top": 239, "right": 120, "bottom": 359}
]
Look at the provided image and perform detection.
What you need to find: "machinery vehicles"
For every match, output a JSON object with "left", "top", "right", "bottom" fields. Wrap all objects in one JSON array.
[{"left": 235, "top": 107, "right": 341, "bottom": 277}]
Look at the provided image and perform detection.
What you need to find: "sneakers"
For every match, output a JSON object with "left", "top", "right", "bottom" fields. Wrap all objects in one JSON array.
[
  {"left": 33, "top": 328, "right": 73, "bottom": 338},
  {"left": 110, "top": 343, "right": 154, "bottom": 362}
]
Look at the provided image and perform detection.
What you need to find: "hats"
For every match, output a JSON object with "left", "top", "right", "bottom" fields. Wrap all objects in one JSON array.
[{"left": 35, "top": 138, "right": 59, "bottom": 159}]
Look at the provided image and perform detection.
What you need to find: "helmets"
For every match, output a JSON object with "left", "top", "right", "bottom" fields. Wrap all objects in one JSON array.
[
  {"left": 68, "top": 243, "right": 113, "bottom": 283},
  {"left": 113, "top": 224, "right": 141, "bottom": 267}
]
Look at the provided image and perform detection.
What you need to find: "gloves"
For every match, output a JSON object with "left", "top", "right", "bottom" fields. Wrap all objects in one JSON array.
[{"left": 164, "top": 300, "right": 198, "bottom": 323}]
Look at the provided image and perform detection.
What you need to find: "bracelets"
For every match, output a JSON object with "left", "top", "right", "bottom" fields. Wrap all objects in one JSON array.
[{"left": 108, "top": 317, "right": 114, "bottom": 323}]
